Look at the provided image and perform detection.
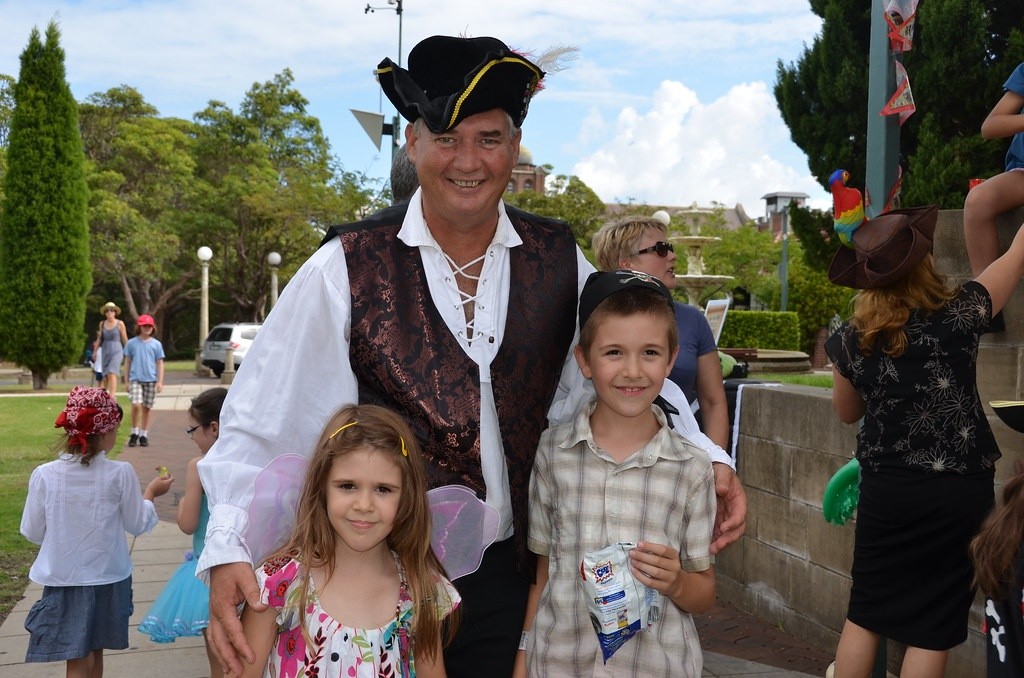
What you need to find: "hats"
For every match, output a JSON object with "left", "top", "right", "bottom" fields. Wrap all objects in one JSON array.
[
  {"left": 137, "top": 315, "right": 154, "bottom": 326},
  {"left": 100, "top": 302, "right": 121, "bottom": 315},
  {"left": 824, "top": 203, "right": 940, "bottom": 292},
  {"left": 577, "top": 269, "right": 676, "bottom": 341},
  {"left": 372, "top": 35, "right": 580, "bottom": 136},
  {"left": 988, "top": 399, "right": 1024, "bottom": 433},
  {"left": 52, "top": 386, "right": 120, "bottom": 454}
]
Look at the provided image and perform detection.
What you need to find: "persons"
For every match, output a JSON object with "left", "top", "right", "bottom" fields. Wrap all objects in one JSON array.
[
  {"left": 123, "top": 315, "right": 166, "bottom": 447},
  {"left": 968, "top": 467, "right": 1024, "bottom": 678},
  {"left": 590, "top": 215, "right": 729, "bottom": 450},
  {"left": 196, "top": 35, "right": 747, "bottom": 678},
  {"left": 963, "top": 62, "right": 1024, "bottom": 333},
  {"left": 138, "top": 387, "right": 228, "bottom": 678},
  {"left": 823, "top": 206, "right": 1024, "bottom": 678},
  {"left": 92, "top": 338, "right": 104, "bottom": 388},
  {"left": 225, "top": 403, "right": 462, "bottom": 678},
  {"left": 20, "top": 386, "right": 173, "bottom": 678},
  {"left": 513, "top": 270, "right": 716, "bottom": 678},
  {"left": 92, "top": 302, "right": 129, "bottom": 397}
]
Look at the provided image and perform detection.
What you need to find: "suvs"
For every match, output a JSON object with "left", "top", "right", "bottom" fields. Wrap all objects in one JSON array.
[{"left": 200, "top": 322, "right": 263, "bottom": 378}]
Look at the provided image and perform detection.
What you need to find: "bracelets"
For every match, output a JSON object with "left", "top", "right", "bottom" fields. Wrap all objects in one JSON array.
[{"left": 518, "top": 630, "right": 530, "bottom": 651}]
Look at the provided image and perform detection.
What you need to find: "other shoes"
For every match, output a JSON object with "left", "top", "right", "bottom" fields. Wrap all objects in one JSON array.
[
  {"left": 139, "top": 436, "right": 147, "bottom": 446},
  {"left": 129, "top": 433, "right": 139, "bottom": 446},
  {"left": 980, "top": 310, "right": 1006, "bottom": 332}
]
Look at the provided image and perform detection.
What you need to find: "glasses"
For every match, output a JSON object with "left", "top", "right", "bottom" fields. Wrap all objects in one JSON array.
[
  {"left": 628, "top": 241, "right": 676, "bottom": 259},
  {"left": 186, "top": 423, "right": 207, "bottom": 438}
]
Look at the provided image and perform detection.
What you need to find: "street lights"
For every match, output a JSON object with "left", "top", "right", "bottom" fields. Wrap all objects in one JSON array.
[
  {"left": 198, "top": 245, "right": 214, "bottom": 378},
  {"left": 268, "top": 251, "right": 283, "bottom": 311}
]
